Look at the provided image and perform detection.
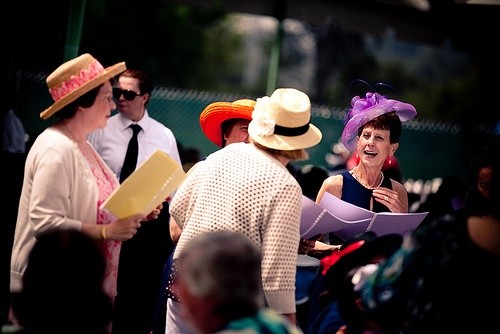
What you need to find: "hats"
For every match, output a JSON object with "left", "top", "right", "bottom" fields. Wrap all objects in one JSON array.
[
  {"left": 40, "top": 53, "right": 127, "bottom": 121},
  {"left": 199, "top": 99, "right": 256, "bottom": 147},
  {"left": 341, "top": 93, "right": 417, "bottom": 153},
  {"left": 248, "top": 88, "right": 322, "bottom": 151}
]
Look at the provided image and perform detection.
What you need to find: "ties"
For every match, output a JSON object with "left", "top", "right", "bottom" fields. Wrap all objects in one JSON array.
[{"left": 118, "top": 123, "right": 142, "bottom": 184}]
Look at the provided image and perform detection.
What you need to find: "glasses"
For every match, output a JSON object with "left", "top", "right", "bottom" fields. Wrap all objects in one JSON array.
[{"left": 112, "top": 87, "right": 144, "bottom": 101}]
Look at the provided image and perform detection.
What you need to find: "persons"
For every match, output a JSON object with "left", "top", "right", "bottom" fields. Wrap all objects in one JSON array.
[
  {"left": 199, "top": 96, "right": 322, "bottom": 256},
  {"left": 164, "top": 86, "right": 324, "bottom": 334},
  {"left": 8, "top": 53, "right": 173, "bottom": 333},
  {"left": 313, "top": 92, "right": 419, "bottom": 220},
  {"left": 327, "top": 162, "right": 500, "bottom": 334},
  {"left": 2, "top": 226, "right": 116, "bottom": 334},
  {"left": 83, "top": 67, "right": 188, "bottom": 334},
  {"left": 173, "top": 229, "right": 305, "bottom": 334},
  {"left": 0, "top": 99, "right": 27, "bottom": 154}
]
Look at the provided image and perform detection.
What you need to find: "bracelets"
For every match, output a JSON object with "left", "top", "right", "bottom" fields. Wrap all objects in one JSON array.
[{"left": 101, "top": 226, "right": 109, "bottom": 241}]
